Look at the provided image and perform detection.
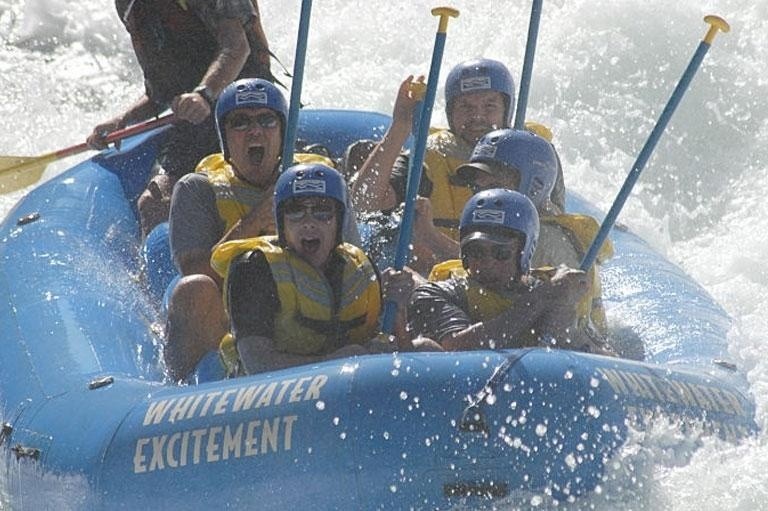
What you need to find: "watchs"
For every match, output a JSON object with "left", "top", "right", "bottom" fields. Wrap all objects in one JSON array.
[{"left": 192, "top": 84, "right": 217, "bottom": 107}]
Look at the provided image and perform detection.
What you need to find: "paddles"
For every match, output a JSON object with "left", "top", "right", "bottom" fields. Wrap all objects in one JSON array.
[{"left": 0, "top": 113, "right": 174, "bottom": 196}]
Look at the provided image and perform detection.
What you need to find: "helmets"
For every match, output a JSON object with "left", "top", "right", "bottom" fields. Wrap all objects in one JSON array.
[
  {"left": 214, "top": 77, "right": 289, "bottom": 158},
  {"left": 455, "top": 128, "right": 559, "bottom": 214},
  {"left": 457, "top": 187, "right": 539, "bottom": 274},
  {"left": 272, "top": 162, "right": 349, "bottom": 249},
  {"left": 444, "top": 56, "right": 516, "bottom": 134}
]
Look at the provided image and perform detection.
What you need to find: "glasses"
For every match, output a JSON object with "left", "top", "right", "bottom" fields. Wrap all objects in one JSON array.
[
  {"left": 285, "top": 204, "right": 336, "bottom": 221},
  {"left": 225, "top": 111, "right": 280, "bottom": 132},
  {"left": 466, "top": 242, "right": 512, "bottom": 261}
]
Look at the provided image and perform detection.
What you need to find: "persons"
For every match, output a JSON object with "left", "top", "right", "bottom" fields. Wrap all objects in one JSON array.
[
  {"left": 219, "top": 161, "right": 415, "bottom": 381},
  {"left": 164, "top": 77, "right": 342, "bottom": 383},
  {"left": 407, "top": 187, "right": 617, "bottom": 363},
  {"left": 411, "top": 126, "right": 609, "bottom": 335},
  {"left": 83, "top": 0, "right": 272, "bottom": 293},
  {"left": 352, "top": 56, "right": 567, "bottom": 243}
]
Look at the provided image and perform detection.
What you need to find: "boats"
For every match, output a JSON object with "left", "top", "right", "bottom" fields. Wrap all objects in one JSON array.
[{"left": 1, "top": 108, "right": 759, "bottom": 510}]
